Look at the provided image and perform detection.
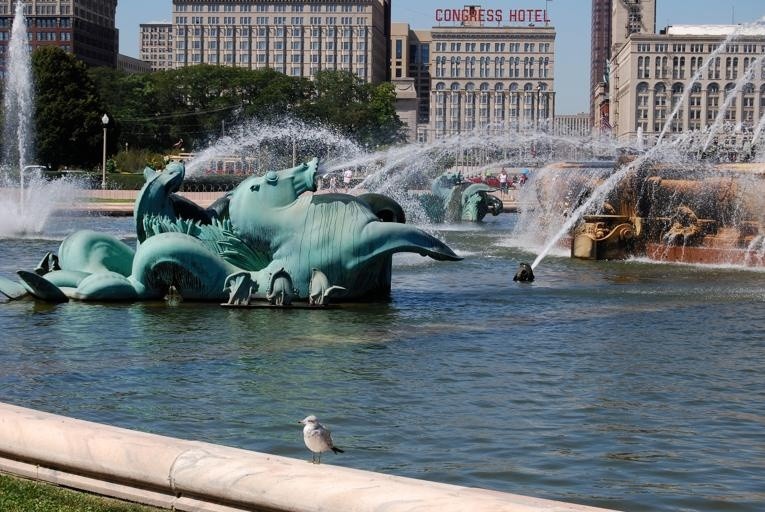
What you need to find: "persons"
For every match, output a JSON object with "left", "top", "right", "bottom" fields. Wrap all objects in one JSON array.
[
  {"left": 319, "top": 172, "right": 326, "bottom": 191},
  {"left": 329, "top": 175, "right": 338, "bottom": 192},
  {"left": 448, "top": 168, "right": 528, "bottom": 198},
  {"left": 342, "top": 166, "right": 352, "bottom": 193}
]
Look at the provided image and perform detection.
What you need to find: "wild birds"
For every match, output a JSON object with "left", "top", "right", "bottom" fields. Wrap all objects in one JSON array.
[{"left": 297, "top": 415, "right": 345, "bottom": 463}]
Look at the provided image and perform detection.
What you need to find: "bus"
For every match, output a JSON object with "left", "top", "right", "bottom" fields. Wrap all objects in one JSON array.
[{"left": 164, "top": 151, "right": 256, "bottom": 175}]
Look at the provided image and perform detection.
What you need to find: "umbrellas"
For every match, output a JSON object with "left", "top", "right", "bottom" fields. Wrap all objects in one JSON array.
[{"left": 519, "top": 168, "right": 529, "bottom": 174}]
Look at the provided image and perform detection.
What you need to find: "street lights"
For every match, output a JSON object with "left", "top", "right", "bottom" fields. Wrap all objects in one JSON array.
[
  {"left": 125, "top": 141, "right": 129, "bottom": 155},
  {"left": 287, "top": 103, "right": 387, "bottom": 178},
  {"left": 98, "top": 114, "right": 110, "bottom": 190}
]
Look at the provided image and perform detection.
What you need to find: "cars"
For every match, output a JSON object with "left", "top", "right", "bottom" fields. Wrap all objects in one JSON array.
[
  {"left": 464, "top": 175, "right": 513, "bottom": 188},
  {"left": 22, "top": 165, "right": 46, "bottom": 173},
  {"left": 58, "top": 166, "right": 83, "bottom": 173}
]
